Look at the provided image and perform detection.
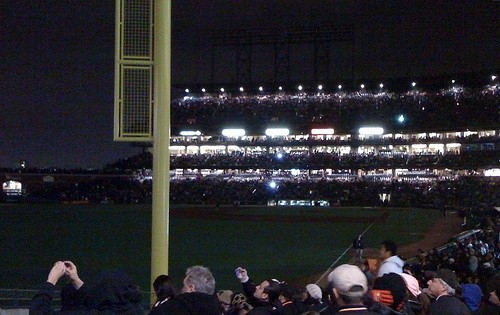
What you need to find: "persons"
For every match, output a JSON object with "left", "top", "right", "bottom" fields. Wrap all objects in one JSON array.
[{"left": 29, "top": 84, "right": 500, "bottom": 315}]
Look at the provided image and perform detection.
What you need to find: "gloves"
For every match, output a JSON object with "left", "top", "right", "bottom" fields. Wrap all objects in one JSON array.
[
  {"left": 395, "top": 297, "right": 409, "bottom": 312},
  {"left": 370, "top": 290, "right": 394, "bottom": 307}
]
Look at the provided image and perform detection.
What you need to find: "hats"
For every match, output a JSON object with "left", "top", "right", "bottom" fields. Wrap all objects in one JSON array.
[
  {"left": 306, "top": 283, "right": 323, "bottom": 302},
  {"left": 373, "top": 273, "right": 406, "bottom": 308},
  {"left": 327, "top": 263, "right": 369, "bottom": 298},
  {"left": 424, "top": 268, "right": 459, "bottom": 290}
]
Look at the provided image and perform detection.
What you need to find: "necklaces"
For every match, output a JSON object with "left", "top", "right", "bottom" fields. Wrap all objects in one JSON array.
[{"left": 281, "top": 299, "right": 294, "bottom": 305}]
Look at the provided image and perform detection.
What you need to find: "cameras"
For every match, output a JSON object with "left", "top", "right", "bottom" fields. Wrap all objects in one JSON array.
[{"left": 236, "top": 270, "right": 242, "bottom": 277}]
